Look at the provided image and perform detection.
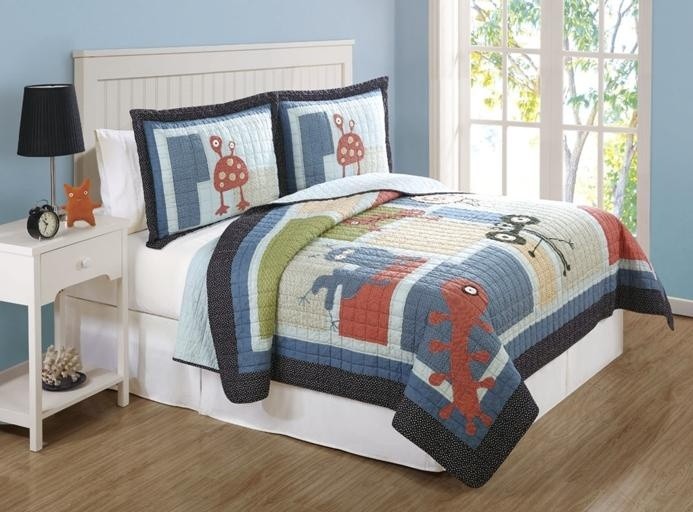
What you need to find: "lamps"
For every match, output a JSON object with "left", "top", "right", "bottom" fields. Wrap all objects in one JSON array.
[{"left": 16, "top": 83, "right": 85, "bottom": 222}]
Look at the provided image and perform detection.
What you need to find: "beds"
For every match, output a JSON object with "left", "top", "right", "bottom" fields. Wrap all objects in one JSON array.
[{"left": 71, "top": 37, "right": 626, "bottom": 476}]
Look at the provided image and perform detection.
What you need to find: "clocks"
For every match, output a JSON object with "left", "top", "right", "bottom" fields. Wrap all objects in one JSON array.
[{"left": 26, "top": 205, "right": 59, "bottom": 240}]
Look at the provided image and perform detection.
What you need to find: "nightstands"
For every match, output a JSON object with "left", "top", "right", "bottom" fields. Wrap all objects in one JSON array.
[{"left": 0, "top": 210, "right": 131, "bottom": 453}]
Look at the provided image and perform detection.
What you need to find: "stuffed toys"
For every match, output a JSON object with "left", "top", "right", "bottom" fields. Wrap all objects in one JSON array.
[{"left": 58, "top": 175, "right": 103, "bottom": 228}]
